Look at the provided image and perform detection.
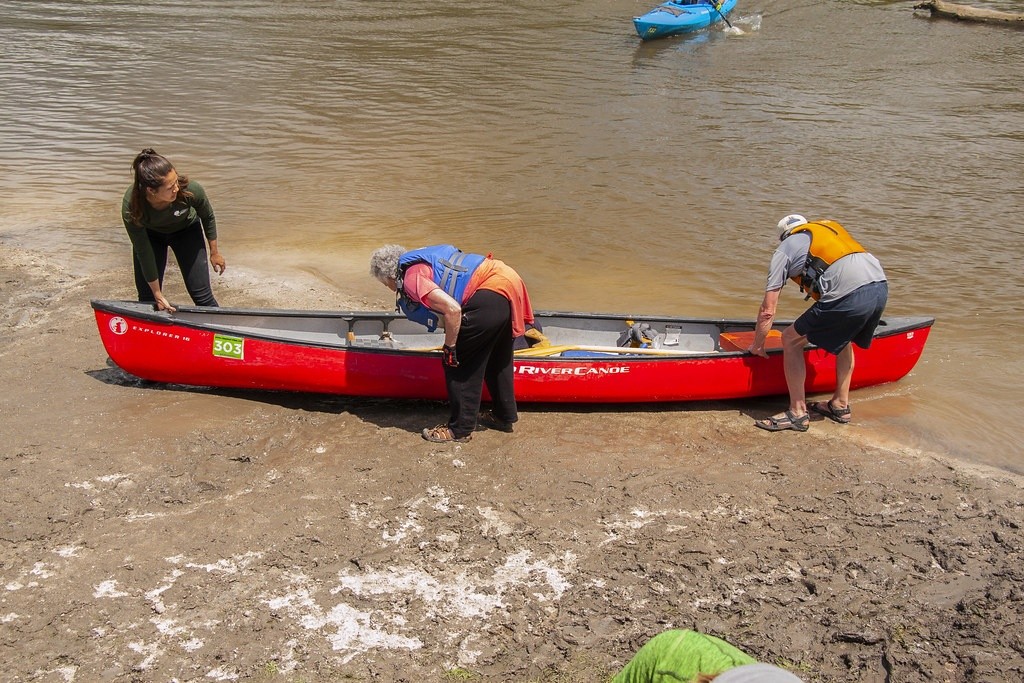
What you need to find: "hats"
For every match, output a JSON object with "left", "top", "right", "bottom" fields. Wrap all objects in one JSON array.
[
  {"left": 709, "top": 662, "right": 804, "bottom": 683},
  {"left": 776, "top": 214, "right": 808, "bottom": 239}
]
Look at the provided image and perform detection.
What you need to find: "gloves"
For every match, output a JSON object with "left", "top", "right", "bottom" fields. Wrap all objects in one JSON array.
[{"left": 441, "top": 343, "right": 459, "bottom": 368}]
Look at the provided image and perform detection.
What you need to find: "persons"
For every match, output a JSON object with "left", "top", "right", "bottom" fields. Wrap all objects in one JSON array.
[
  {"left": 121, "top": 149, "right": 228, "bottom": 313},
  {"left": 674, "top": 0, "right": 726, "bottom": 11},
  {"left": 747, "top": 215, "right": 889, "bottom": 432},
  {"left": 368, "top": 243, "right": 536, "bottom": 444},
  {"left": 606, "top": 628, "right": 804, "bottom": 683}
]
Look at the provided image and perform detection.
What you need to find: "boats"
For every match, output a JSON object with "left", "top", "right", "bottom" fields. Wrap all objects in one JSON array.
[
  {"left": 90, "top": 299, "right": 936, "bottom": 405},
  {"left": 634, "top": 2, "right": 740, "bottom": 37}
]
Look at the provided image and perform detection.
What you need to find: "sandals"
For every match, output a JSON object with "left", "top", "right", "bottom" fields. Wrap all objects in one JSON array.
[
  {"left": 479, "top": 409, "right": 518, "bottom": 425},
  {"left": 805, "top": 399, "right": 851, "bottom": 423},
  {"left": 422, "top": 423, "right": 473, "bottom": 443},
  {"left": 755, "top": 409, "right": 810, "bottom": 431}
]
